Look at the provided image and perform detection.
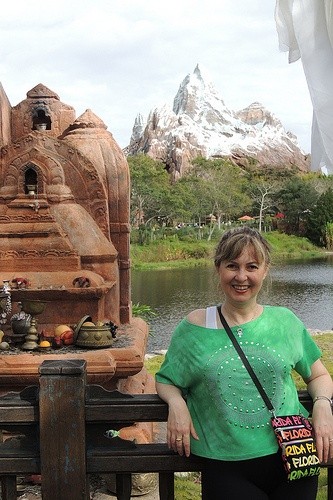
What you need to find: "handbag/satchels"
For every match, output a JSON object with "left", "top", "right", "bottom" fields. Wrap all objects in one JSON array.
[{"left": 271, "top": 414, "right": 321, "bottom": 482}]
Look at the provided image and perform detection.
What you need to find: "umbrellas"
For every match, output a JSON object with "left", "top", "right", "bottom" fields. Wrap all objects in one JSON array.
[{"left": 238, "top": 215, "right": 253, "bottom": 220}]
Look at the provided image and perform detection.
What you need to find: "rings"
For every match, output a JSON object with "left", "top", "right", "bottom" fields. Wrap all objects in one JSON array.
[{"left": 176, "top": 439, "right": 182, "bottom": 441}]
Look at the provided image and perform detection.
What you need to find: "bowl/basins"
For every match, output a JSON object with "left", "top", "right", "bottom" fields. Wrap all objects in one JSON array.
[
  {"left": 34, "top": 123, "right": 48, "bottom": 130},
  {"left": 26, "top": 184, "right": 37, "bottom": 190},
  {"left": 77, "top": 326, "right": 113, "bottom": 347}
]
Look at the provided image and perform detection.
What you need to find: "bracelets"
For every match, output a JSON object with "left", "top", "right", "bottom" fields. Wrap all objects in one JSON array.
[{"left": 313, "top": 395, "right": 332, "bottom": 405}]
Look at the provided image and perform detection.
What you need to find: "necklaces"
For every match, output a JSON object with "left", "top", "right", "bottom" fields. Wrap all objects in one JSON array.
[{"left": 222, "top": 307, "right": 258, "bottom": 338}]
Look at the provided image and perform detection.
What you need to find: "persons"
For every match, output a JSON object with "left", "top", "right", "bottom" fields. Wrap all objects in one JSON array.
[{"left": 156, "top": 226, "right": 333, "bottom": 500}]
